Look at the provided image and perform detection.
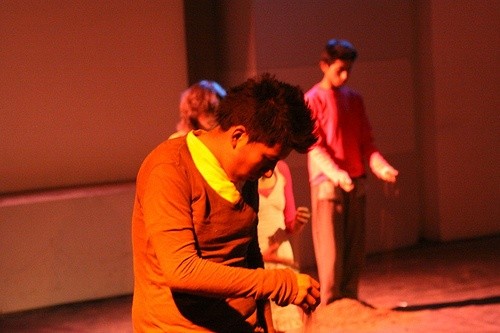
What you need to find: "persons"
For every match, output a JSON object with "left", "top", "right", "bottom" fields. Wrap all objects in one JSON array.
[
  {"left": 167, "top": 82, "right": 312, "bottom": 333},
  {"left": 131, "top": 71, "right": 322, "bottom": 333},
  {"left": 304, "top": 39, "right": 398, "bottom": 309}
]
